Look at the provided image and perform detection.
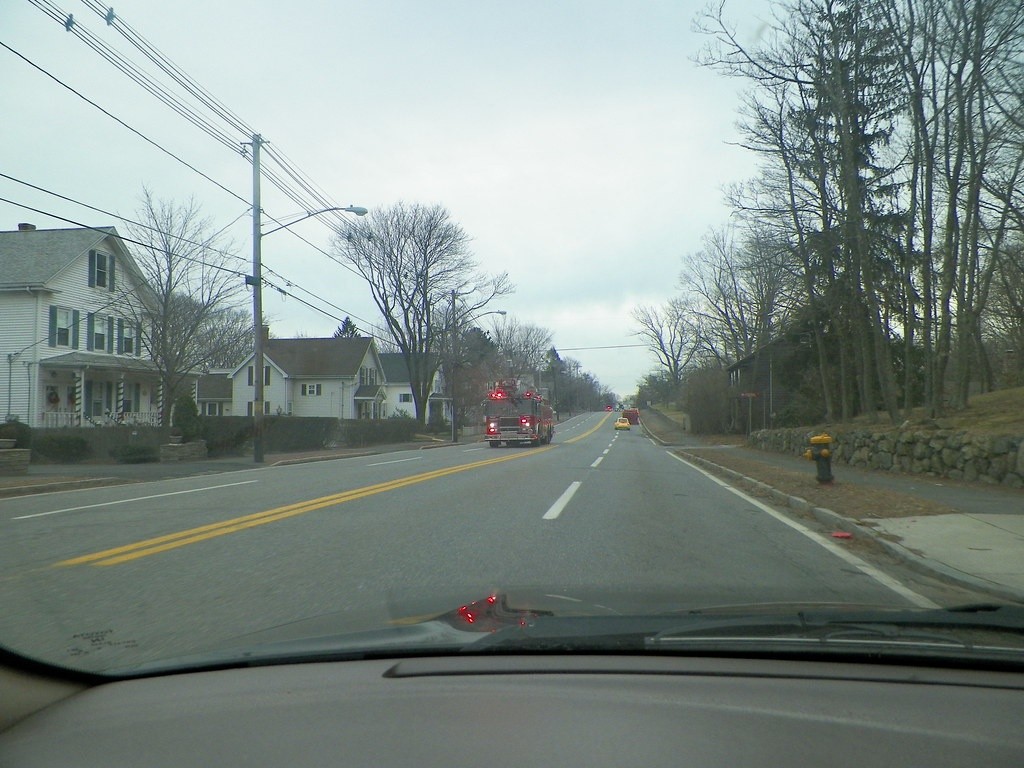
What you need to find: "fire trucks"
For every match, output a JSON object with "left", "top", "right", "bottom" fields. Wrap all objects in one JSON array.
[
  {"left": 483, "top": 380, "right": 555, "bottom": 448},
  {"left": 622, "top": 409, "right": 640, "bottom": 425}
]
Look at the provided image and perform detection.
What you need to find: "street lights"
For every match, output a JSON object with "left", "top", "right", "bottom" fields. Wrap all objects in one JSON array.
[
  {"left": 252, "top": 205, "right": 368, "bottom": 460},
  {"left": 451, "top": 311, "right": 508, "bottom": 442}
]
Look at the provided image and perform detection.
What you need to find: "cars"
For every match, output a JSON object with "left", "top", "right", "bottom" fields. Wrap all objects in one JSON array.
[
  {"left": 614, "top": 417, "right": 631, "bottom": 431},
  {"left": 605, "top": 405, "right": 612, "bottom": 412}
]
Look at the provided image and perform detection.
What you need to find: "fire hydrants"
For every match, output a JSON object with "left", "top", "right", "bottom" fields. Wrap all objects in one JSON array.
[{"left": 805, "top": 433, "right": 836, "bottom": 483}]
